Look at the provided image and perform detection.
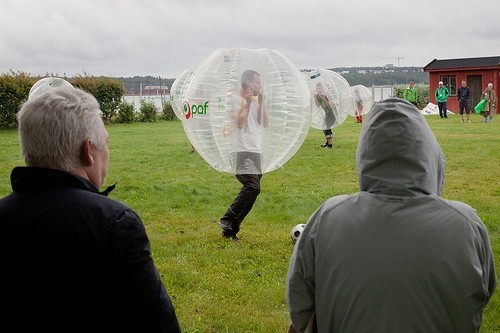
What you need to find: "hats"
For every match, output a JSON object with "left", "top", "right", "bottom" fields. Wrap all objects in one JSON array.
[{"left": 439, "top": 81, "right": 443, "bottom": 85}]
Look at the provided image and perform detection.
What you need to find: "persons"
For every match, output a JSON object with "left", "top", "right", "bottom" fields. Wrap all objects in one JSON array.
[
  {"left": 354, "top": 90, "right": 365, "bottom": 123},
  {"left": 403, "top": 81, "right": 418, "bottom": 106},
  {"left": 312, "top": 82, "right": 338, "bottom": 148},
  {"left": 434, "top": 81, "right": 450, "bottom": 118},
  {"left": 218, "top": 69, "right": 270, "bottom": 240},
  {"left": 286, "top": 97, "right": 497, "bottom": 333},
  {"left": 481, "top": 83, "right": 496, "bottom": 124},
  {"left": 0, "top": 88, "right": 182, "bottom": 333},
  {"left": 457, "top": 80, "right": 471, "bottom": 124}
]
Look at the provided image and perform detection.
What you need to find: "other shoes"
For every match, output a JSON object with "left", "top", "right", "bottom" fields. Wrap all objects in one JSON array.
[
  {"left": 219, "top": 219, "right": 232, "bottom": 231},
  {"left": 467, "top": 119, "right": 470, "bottom": 123},
  {"left": 461, "top": 120, "right": 464, "bottom": 123},
  {"left": 222, "top": 229, "right": 239, "bottom": 241}
]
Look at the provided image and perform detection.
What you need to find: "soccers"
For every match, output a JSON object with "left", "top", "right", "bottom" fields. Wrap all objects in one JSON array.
[{"left": 290, "top": 223, "right": 307, "bottom": 246}]
